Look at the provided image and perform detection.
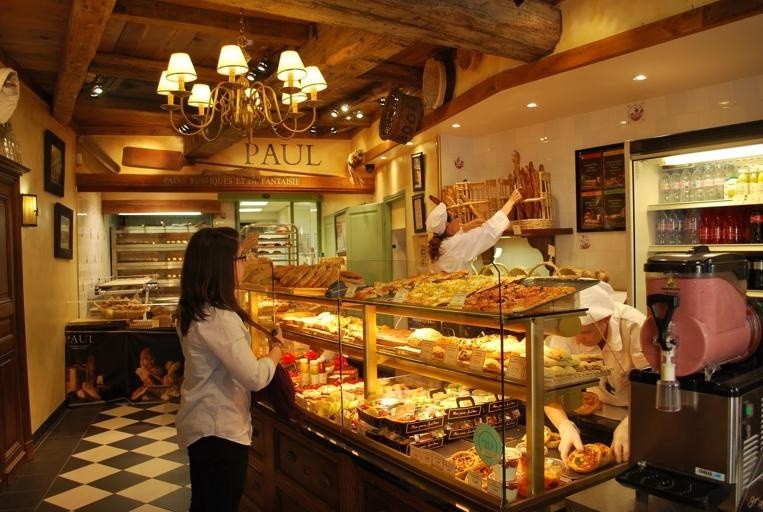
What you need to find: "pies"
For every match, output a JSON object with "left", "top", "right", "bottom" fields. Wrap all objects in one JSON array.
[
  {"left": 547, "top": 432, "right": 562, "bottom": 448},
  {"left": 567, "top": 444, "right": 602, "bottom": 473},
  {"left": 596, "top": 443, "right": 612, "bottom": 467},
  {"left": 280, "top": 265, "right": 360, "bottom": 288},
  {"left": 522, "top": 425, "right": 552, "bottom": 445},
  {"left": 449, "top": 446, "right": 492, "bottom": 488},
  {"left": 516, "top": 442, "right": 549, "bottom": 459}
]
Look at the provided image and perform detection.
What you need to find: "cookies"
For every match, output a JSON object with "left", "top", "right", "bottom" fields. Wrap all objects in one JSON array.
[{"left": 301, "top": 382, "right": 357, "bottom": 418}]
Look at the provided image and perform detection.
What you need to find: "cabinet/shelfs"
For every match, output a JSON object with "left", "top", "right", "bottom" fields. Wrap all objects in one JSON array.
[
  {"left": 232, "top": 276, "right": 633, "bottom": 512},
  {"left": 242, "top": 224, "right": 299, "bottom": 267},
  {"left": 99, "top": 194, "right": 214, "bottom": 320}
]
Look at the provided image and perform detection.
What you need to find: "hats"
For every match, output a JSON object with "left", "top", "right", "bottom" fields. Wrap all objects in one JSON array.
[
  {"left": 575, "top": 277, "right": 621, "bottom": 323},
  {"left": 428, "top": 202, "right": 448, "bottom": 237}
]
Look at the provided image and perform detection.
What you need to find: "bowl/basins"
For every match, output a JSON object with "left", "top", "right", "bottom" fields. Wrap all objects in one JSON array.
[{"left": 487, "top": 441, "right": 565, "bottom": 504}]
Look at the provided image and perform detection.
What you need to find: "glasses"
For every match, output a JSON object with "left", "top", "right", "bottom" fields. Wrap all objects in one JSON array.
[{"left": 231, "top": 254, "right": 246, "bottom": 261}]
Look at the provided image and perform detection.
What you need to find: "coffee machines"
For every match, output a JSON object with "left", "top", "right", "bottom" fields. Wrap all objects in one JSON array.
[{"left": 613, "top": 246, "right": 763, "bottom": 512}]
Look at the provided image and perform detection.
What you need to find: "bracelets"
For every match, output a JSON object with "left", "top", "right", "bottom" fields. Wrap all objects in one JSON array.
[{"left": 269, "top": 341, "right": 286, "bottom": 357}]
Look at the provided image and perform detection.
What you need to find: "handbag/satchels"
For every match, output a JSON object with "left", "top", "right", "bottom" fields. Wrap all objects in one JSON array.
[{"left": 250, "top": 363, "right": 297, "bottom": 416}]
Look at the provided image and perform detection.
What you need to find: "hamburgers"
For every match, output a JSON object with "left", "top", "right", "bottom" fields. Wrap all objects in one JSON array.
[{"left": 358, "top": 383, "right": 520, "bottom": 445}]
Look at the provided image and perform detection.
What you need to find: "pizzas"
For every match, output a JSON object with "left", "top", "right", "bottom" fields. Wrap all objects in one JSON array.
[
  {"left": 355, "top": 271, "right": 468, "bottom": 299},
  {"left": 463, "top": 283, "right": 575, "bottom": 313},
  {"left": 403, "top": 275, "right": 504, "bottom": 307}
]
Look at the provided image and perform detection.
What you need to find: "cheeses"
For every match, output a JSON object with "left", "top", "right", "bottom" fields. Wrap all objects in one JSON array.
[
  {"left": 494, "top": 448, "right": 520, "bottom": 481},
  {"left": 489, "top": 473, "right": 519, "bottom": 503}
]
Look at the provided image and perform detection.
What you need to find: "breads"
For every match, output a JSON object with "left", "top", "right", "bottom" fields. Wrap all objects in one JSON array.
[
  {"left": 272, "top": 307, "right": 364, "bottom": 344},
  {"left": 257, "top": 301, "right": 290, "bottom": 328},
  {"left": 375, "top": 327, "right": 527, "bottom": 375},
  {"left": 544, "top": 345, "right": 604, "bottom": 376}
]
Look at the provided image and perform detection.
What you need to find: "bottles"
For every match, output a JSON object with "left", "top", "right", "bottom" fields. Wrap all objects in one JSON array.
[
  {"left": 298, "top": 357, "right": 328, "bottom": 386},
  {"left": 659, "top": 161, "right": 763, "bottom": 203},
  {"left": 655, "top": 205, "right": 763, "bottom": 245}
]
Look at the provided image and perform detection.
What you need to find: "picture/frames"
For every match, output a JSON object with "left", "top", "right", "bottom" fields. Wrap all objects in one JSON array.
[
  {"left": 44, "top": 129, "right": 67, "bottom": 199},
  {"left": 412, "top": 193, "right": 426, "bottom": 234},
  {"left": 53, "top": 202, "right": 74, "bottom": 260},
  {"left": 411, "top": 151, "right": 424, "bottom": 191}
]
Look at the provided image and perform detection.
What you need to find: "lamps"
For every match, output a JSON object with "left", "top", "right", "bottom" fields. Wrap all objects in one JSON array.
[
  {"left": 22, "top": 193, "right": 38, "bottom": 225},
  {"left": 157, "top": 7, "right": 328, "bottom": 143},
  {"left": 246, "top": 57, "right": 270, "bottom": 79},
  {"left": 90, "top": 76, "right": 103, "bottom": 98}
]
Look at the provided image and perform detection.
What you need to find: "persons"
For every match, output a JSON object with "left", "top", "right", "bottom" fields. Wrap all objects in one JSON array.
[
  {"left": 425, "top": 188, "right": 523, "bottom": 336},
  {"left": 172, "top": 227, "right": 285, "bottom": 512},
  {"left": 541, "top": 278, "right": 653, "bottom": 465}
]
